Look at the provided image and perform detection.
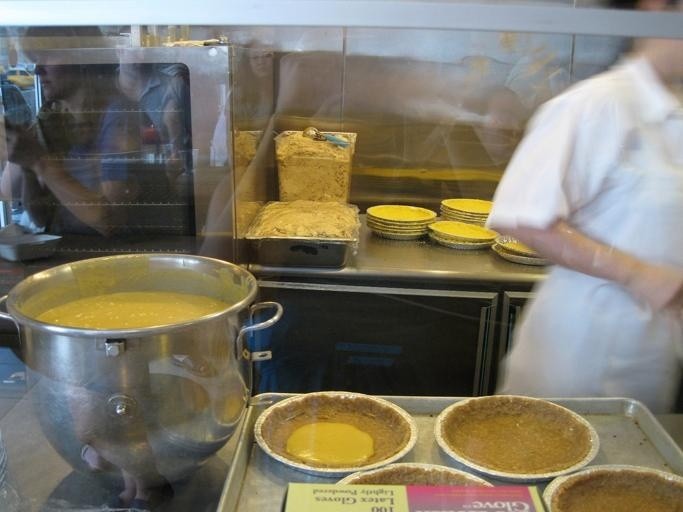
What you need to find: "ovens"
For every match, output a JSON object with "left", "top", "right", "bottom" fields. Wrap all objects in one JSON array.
[{"left": 10, "top": 35, "right": 276, "bottom": 267}]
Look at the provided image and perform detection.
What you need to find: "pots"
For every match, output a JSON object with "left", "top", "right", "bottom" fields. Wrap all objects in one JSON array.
[{"left": 4, "top": 248, "right": 283, "bottom": 491}]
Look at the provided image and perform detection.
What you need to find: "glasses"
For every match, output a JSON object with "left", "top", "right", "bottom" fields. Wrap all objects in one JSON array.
[{"left": 248, "top": 52, "right": 272, "bottom": 61}]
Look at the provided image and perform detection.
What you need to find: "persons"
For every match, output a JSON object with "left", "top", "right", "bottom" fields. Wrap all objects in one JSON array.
[
  {"left": 1, "top": 25, "right": 317, "bottom": 263},
  {"left": 481, "top": 0, "right": 683, "bottom": 413}
]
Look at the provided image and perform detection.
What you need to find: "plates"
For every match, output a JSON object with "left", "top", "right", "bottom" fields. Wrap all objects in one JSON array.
[
  {"left": 255, "top": 392, "right": 418, "bottom": 476},
  {"left": 331, "top": 464, "right": 495, "bottom": 487},
  {"left": 367, "top": 198, "right": 548, "bottom": 268},
  {"left": 217, "top": 394, "right": 683, "bottom": 511},
  {"left": 540, "top": 461, "right": 683, "bottom": 512},
  {"left": 430, "top": 394, "right": 600, "bottom": 482}
]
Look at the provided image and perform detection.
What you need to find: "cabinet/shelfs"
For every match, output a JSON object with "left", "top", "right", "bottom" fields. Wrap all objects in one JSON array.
[{"left": 254, "top": 281, "right": 534, "bottom": 396}]
